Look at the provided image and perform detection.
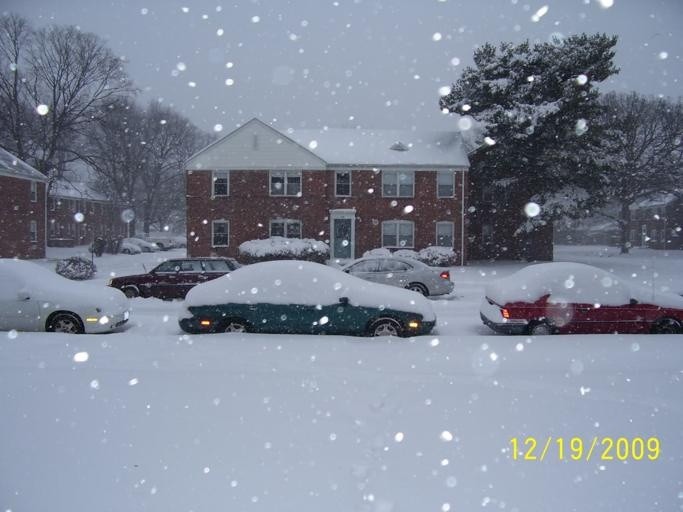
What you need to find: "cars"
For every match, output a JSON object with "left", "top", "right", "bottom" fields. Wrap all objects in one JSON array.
[
  {"left": 179, "top": 297, "right": 436, "bottom": 337},
  {"left": 89, "top": 233, "right": 188, "bottom": 254},
  {"left": 0, "top": 288, "right": 131, "bottom": 334},
  {"left": 109, "top": 257, "right": 242, "bottom": 298},
  {"left": 341, "top": 255, "right": 454, "bottom": 297},
  {"left": 480, "top": 295, "right": 683, "bottom": 335}
]
